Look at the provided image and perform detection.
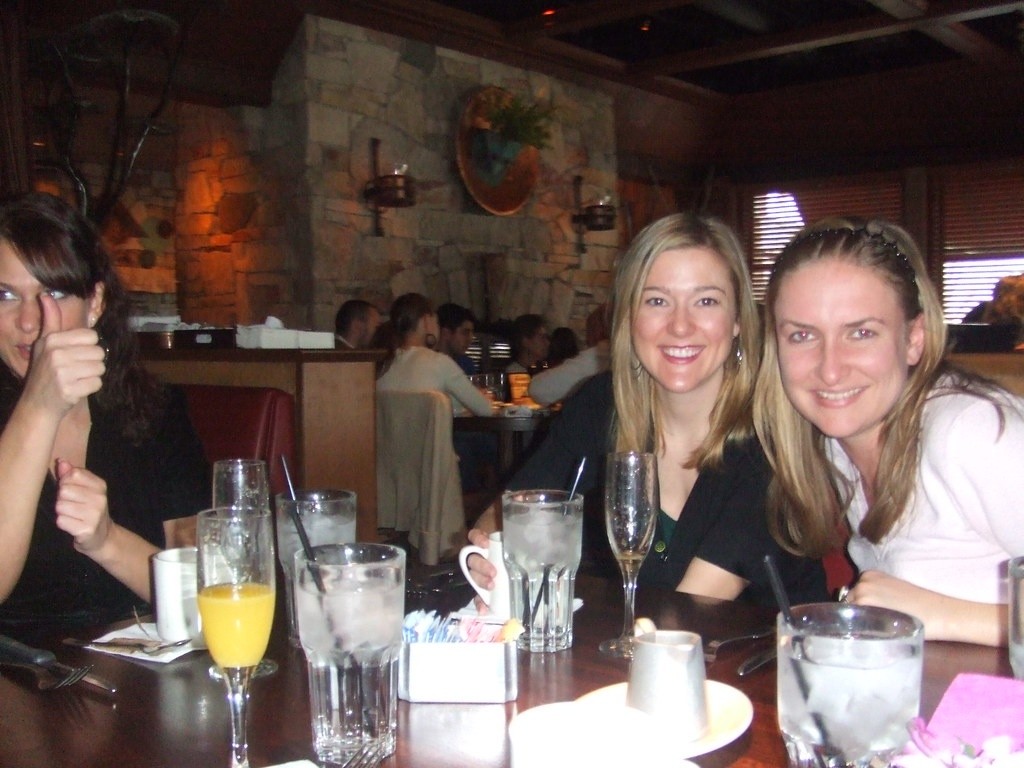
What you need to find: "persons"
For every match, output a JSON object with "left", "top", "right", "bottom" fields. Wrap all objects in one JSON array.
[
  {"left": 467, "top": 208, "right": 833, "bottom": 613},
  {"left": 0, "top": 189, "right": 213, "bottom": 620},
  {"left": 750, "top": 212, "right": 1024, "bottom": 646},
  {"left": 952, "top": 274, "right": 1024, "bottom": 355},
  {"left": 333, "top": 291, "right": 620, "bottom": 566}
]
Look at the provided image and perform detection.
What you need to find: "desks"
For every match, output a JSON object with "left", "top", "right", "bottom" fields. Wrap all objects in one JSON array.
[
  {"left": 0, "top": 540, "right": 1013, "bottom": 767},
  {"left": 444, "top": 408, "right": 555, "bottom": 488}
]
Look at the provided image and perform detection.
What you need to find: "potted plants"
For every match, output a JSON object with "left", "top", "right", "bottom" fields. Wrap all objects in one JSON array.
[{"left": 466, "top": 96, "right": 557, "bottom": 186}]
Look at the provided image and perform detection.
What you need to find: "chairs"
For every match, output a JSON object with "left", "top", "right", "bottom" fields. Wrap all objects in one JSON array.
[
  {"left": 376, "top": 389, "right": 469, "bottom": 568},
  {"left": 173, "top": 382, "right": 295, "bottom": 504}
]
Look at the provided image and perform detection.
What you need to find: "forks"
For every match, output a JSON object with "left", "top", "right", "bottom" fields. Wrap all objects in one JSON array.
[{"left": 1, "top": 657, "right": 94, "bottom": 692}]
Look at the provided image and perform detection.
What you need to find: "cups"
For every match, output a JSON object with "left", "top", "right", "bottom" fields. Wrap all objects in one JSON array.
[
  {"left": 459, "top": 490, "right": 583, "bottom": 652},
  {"left": 624, "top": 618, "right": 709, "bottom": 743},
  {"left": 776, "top": 556, "right": 1024, "bottom": 768},
  {"left": 209, "top": 459, "right": 278, "bottom": 680},
  {"left": 508, "top": 699, "right": 700, "bottom": 768},
  {"left": 467, "top": 373, "right": 504, "bottom": 403},
  {"left": 274, "top": 488, "right": 406, "bottom": 767},
  {"left": 151, "top": 545, "right": 201, "bottom": 643}
]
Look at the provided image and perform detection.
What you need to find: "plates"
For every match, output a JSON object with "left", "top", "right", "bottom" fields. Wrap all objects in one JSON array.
[{"left": 575, "top": 681, "right": 755, "bottom": 758}]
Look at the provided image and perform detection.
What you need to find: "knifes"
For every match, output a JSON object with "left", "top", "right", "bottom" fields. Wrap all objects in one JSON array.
[
  {"left": 737, "top": 646, "right": 776, "bottom": 676},
  {"left": 0, "top": 634, "right": 117, "bottom": 693}
]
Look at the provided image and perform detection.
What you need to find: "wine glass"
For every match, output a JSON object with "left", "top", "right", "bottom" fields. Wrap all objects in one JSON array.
[
  {"left": 197, "top": 510, "right": 277, "bottom": 768},
  {"left": 599, "top": 453, "right": 658, "bottom": 660}
]
